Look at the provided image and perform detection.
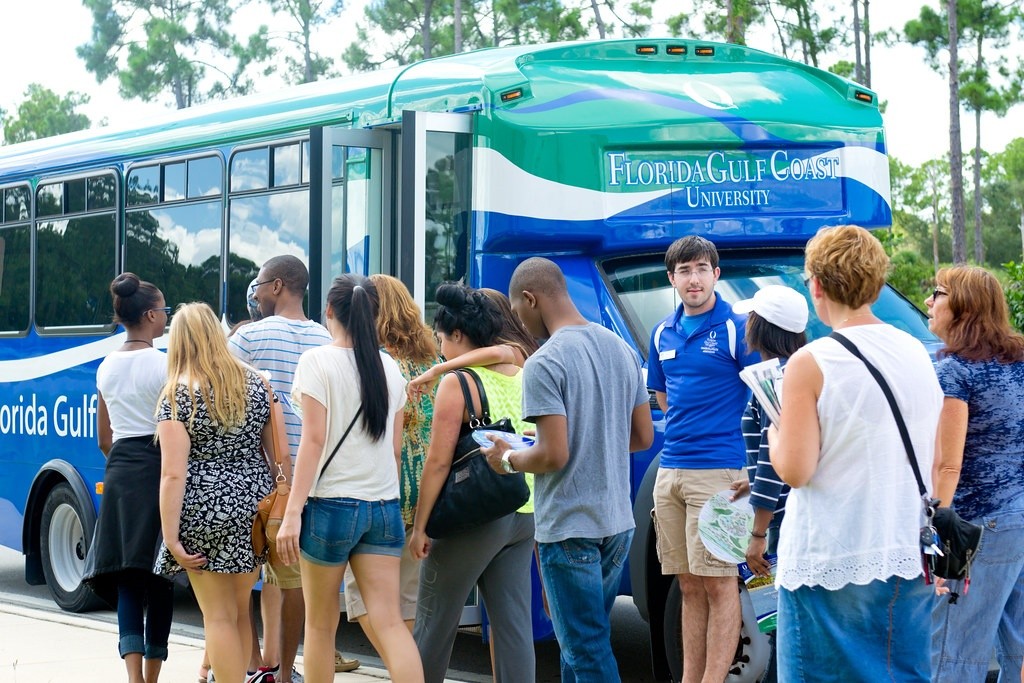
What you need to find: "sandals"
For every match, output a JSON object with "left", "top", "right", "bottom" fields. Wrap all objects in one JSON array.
[{"left": 198, "top": 664, "right": 211, "bottom": 683}]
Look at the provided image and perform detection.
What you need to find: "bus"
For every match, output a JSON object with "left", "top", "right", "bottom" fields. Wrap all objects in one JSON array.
[{"left": 1, "top": 36, "right": 948, "bottom": 683}]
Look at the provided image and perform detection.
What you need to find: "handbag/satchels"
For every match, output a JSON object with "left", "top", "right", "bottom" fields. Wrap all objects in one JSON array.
[
  {"left": 925, "top": 500, "right": 985, "bottom": 582},
  {"left": 423, "top": 370, "right": 531, "bottom": 538},
  {"left": 251, "top": 482, "right": 293, "bottom": 566}
]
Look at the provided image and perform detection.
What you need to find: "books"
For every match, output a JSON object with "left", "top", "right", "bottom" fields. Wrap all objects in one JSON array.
[
  {"left": 738, "top": 552, "right": 778, "bottom": 631},
  {"left": 761, "top": 380, "right": 781, "bottom": 416}
]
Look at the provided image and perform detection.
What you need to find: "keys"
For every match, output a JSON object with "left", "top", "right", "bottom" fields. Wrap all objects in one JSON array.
[{"left": 922, "top": 535, "right": 944, "bottom": 557}]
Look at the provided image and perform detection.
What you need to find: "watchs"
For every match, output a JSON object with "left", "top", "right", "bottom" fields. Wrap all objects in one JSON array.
[{"left": 502, "top": 450, "right": 520, "bottom": 473}]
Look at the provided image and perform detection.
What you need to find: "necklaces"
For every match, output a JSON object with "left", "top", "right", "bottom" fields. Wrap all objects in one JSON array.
[
  {"left": 844, "top": 314, "right": 873, "bottom": 321},
  {"left": 124, "top": 340, "right": 153, "bottom": 347}
]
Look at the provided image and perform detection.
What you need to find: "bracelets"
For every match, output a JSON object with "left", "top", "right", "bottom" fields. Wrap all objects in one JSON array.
[{"left": 751, "top": 533, "right": 767, "bottom": 537}]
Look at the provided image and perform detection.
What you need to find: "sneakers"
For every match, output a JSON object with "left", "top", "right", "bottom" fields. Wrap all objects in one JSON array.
[
  {"left": 270, "top": 664, "right": 304, "bottom": 683},
  {"left": 245, "top": 666, "right": 274, "bottom": 683},
  {"left": 333, "top": 648, "right": 360, "bottom": 672}
]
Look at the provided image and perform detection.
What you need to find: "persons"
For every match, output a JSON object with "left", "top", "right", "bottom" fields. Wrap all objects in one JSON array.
[
  {"left": 276, "top": 276, "right": 425, "bottom": 683},
  {"left": 345, "top": 274, "right": 445, "bottom": 676},
  {"left": 97, "top": 272, "right": 174, "bottom": 683},
  {"left": 646, "top": 236, "right": 763, "bottom": 682},
  {"left": 735, "top": 285, "right": 809, "bottom": 579},
  {"left": 411, "top": 287, "right": 553, "bottom": 683},
  {"left": 481, "top": 257, "right": 655, "bottom": 682},
  {"left": 925, "top": 267, "right": 1024, "bottom": 683},
  {"left": 767, "top": 224, "right": 944, "bottom": 683},
  {"left": 198, "top": 255, "right": 361, "bottom": 683},
  {"left": 154, "top": 299, "right": 292, "bottom": 683}
]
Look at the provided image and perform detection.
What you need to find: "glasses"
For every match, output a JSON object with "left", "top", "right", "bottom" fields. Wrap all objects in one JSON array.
[
  {"left": 672, "top": 268, "right": 715, "bottom": 281},
  {"left": 143, "top": 306, "right": 171, "bottom": 316},
  {"left": 251, "top": 281, "right": 275, "bottom": 293},
  {"left": 933, "top": 289, "right": 950, "bottom": 303},
  {"left": 803, "top": 274, "right": 816, "bottom": 288}
]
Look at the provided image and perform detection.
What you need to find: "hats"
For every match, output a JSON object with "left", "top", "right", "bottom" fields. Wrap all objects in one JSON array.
[{"left": 731, "top": 285, "right": 808, "bottom": 333}]
